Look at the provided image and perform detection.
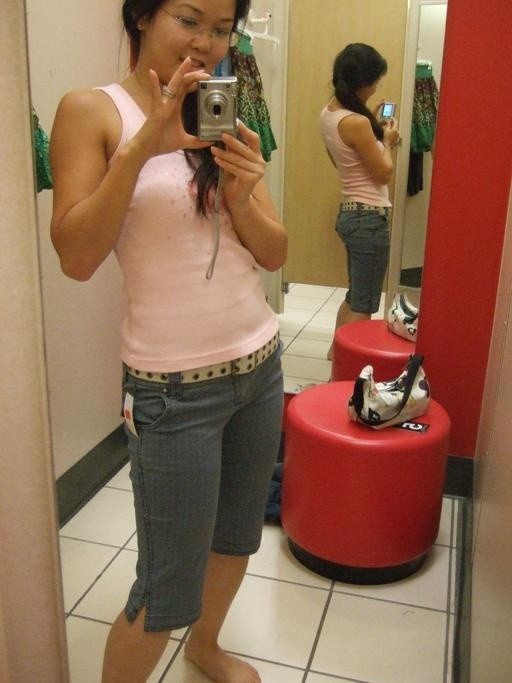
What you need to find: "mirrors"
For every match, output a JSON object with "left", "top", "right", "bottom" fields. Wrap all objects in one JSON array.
[{"left": 271, "top": 1, "right": 448, "bottom": 396}]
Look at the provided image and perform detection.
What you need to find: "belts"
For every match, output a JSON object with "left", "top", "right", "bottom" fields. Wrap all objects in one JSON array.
[
  {"left": 127, "top": 332, "right": 279, "bottom": 384},
  {"left": 341, "top": 202, "right": 390, "bottom": 216}
]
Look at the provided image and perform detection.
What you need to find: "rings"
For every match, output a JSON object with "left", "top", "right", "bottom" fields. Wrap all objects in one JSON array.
[{"left": 160, "top": 85, "right": 175, "bottom": 100}]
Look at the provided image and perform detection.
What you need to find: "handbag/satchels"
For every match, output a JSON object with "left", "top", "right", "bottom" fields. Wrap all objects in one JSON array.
[
  {"left": 348, "top": 353, "right": 431, "bottom": 429},
  {"left": 387, "top": 290, "right": 419, "bottom": 342}
]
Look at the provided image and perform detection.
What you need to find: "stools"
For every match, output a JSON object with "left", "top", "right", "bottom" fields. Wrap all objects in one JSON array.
[
  {"left": 332, "top": 319, "right": 419, "bottom": 382},
  {"left": 285, "top": 382, "right": 452, "bottom": 586}
]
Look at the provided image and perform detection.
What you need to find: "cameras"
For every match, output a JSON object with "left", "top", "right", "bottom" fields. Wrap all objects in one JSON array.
[
  {"left": 193, "top": 74, "right": 239, "bottom": 144},
  {"left": 381, "top": 102, "right": 398, "bottom": 125}
]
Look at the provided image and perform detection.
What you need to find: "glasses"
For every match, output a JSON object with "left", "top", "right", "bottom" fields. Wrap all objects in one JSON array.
[{"left": 158, "top": 7, "right": 239, "bottom": 47}]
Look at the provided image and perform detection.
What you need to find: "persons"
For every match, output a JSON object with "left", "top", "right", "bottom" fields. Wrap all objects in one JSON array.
[
  {"left": 317, "top": 42, "right": 401, "bottom": 384},
  {"left": 48, "top": 0, "right": 293, "bottom": 683}
]
